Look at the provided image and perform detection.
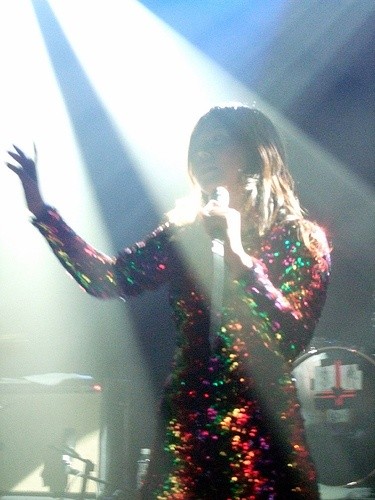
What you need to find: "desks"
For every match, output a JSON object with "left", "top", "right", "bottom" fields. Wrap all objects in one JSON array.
[{"left": 1, "top": 377, "right": 130, "bottom": 498}]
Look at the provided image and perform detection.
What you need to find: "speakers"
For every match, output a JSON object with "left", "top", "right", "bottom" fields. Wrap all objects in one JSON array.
[{"left": 0, "top": 383, "right": 109, "bottom": 500}]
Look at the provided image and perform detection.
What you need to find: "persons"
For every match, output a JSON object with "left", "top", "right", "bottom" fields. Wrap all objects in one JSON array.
[{"left": 6, "top": 103, "right": 331, "bottom": 500}]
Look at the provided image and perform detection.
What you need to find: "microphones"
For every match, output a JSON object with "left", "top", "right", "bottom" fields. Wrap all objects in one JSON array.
[
  {"left": 59, "top": 443, "right": 80, "bottom": 459},
  {"left": 209, "top": 185, "right": 229, "bottom": 256}
]
